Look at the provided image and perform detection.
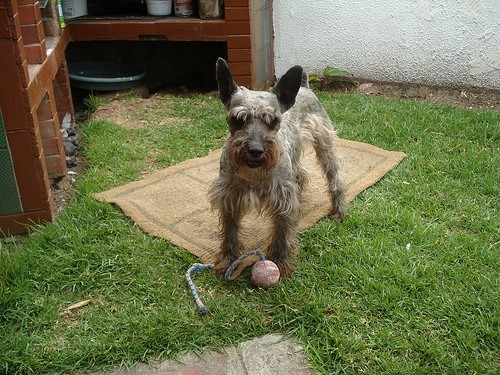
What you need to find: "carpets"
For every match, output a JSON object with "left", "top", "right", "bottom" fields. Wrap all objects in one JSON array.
[{"left": 90, "top": 139, "right": 408, "bottom": 280}]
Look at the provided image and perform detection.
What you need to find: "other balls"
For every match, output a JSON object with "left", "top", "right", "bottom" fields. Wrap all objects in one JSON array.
[{"left": 251, "top": 260, "right": 280, "bottom": 288}]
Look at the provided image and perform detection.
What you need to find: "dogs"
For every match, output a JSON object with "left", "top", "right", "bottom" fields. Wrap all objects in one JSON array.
[{"left": 206, "top": 58, "right": 351, "bottom": 280}]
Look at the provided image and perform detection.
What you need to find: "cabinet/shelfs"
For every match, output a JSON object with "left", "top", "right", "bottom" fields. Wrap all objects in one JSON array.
[{"left": 0, "top": 0, "right": 276, "bottom": 239}]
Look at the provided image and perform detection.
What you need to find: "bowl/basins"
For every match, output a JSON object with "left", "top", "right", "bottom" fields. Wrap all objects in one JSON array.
[
  {"left": 146, "top": 0, "right": 172, "bottom": 16},
  {"left": 68, "top": 60, "right": 147, "bottom": 90}
]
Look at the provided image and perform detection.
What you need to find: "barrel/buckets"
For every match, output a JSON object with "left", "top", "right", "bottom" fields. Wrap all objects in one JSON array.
[
  {"left": 62, "top": 0, "right": 88, "bottom": 19},
  {"left": 199, "top": 0, "right": 221, "bottom": 19},
  {"left": 174, "top": 0, "right": 193, "bottom": 17}
]
